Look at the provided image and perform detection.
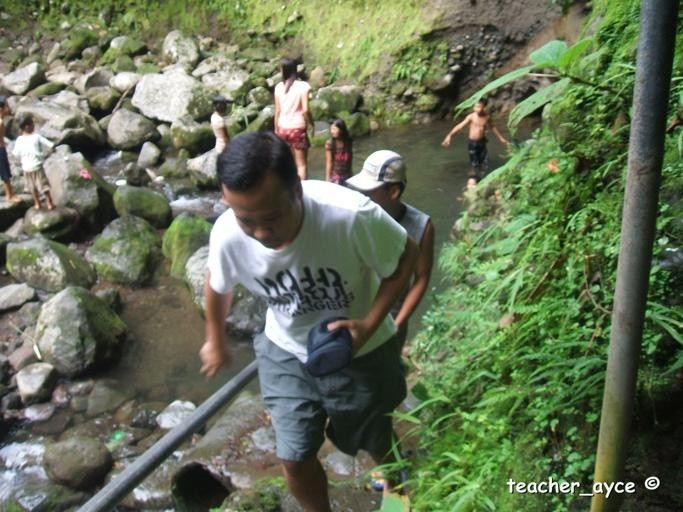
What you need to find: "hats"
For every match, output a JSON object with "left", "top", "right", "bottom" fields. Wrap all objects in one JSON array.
[
  {"left": 344, "top": 148, "right": 407, "bottom": 192},
  {"left": 211, "top": 93, "right": 235, "bottom": 103}
]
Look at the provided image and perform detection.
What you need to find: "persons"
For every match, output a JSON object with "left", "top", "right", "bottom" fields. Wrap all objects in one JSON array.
[
  {"left": 344, "top": 149, "right": 435, "bottom": 491},
  {"left": 466, "top": 175, "right": 481, "bottom": 190},
  {"left": 440, "top": 98, "right": 511, "bottom": 178},
  {"left": 325, "top": 118, "right": 354, "bottom": 187},
  {"left": 273, "top": 58, "right": 315, "bottom": 181},
  {"left": 198, "top": 130, "right": 420, "bottom": 512},
  {"left": 210, "top": 95, "right": 232, "bottom": 208}
]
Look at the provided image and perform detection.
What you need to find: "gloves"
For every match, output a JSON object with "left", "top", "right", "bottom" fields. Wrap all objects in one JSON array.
[{"left": 305, "top": 316, "right": 352, "bottom": 376}]
